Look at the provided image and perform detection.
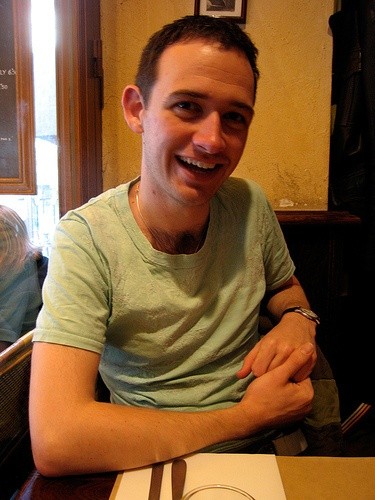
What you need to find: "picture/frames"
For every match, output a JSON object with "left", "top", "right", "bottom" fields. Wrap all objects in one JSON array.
[
  {"left": 194, "top": 0, "right": 247, "bottom": 24},
  {"left": 0, "top": 0, "right": 36, "bottom": 195}
]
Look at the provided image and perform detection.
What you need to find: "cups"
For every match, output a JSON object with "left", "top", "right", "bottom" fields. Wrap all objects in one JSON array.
[{"left": 182, "top": 485, "right": 255, "bottom": 500}]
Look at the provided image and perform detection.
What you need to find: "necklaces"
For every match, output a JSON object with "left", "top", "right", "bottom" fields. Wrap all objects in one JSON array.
[{"left": 136, "top": 184, "right": 207, "bottom": 254}]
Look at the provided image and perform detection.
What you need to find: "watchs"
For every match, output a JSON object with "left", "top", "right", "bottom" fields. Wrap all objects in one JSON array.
[{"left": 281, "top": 306, "right": 321, "bottom": 325}]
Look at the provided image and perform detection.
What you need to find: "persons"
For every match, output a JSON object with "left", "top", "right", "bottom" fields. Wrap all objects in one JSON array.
[
  {"left": 29, "top": 15, "right": 322, "bottom": 476},
  {"left": 0, "top": 205, "right": 42, "bottom": 352}
]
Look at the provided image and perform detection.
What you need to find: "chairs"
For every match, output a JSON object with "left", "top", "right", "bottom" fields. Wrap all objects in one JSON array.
[{"left": 0, "top": 315, "right": 342, "bottom": 500}]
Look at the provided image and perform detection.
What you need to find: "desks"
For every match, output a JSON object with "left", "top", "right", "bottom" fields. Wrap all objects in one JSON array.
[{"left": 273, "top": 210, "right": 360, "bottom": 298}]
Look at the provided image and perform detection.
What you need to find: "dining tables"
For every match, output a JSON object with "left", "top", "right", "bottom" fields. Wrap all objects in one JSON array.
[{"left": 109, "top": 452, "right": 375, "bottom": 500}]
